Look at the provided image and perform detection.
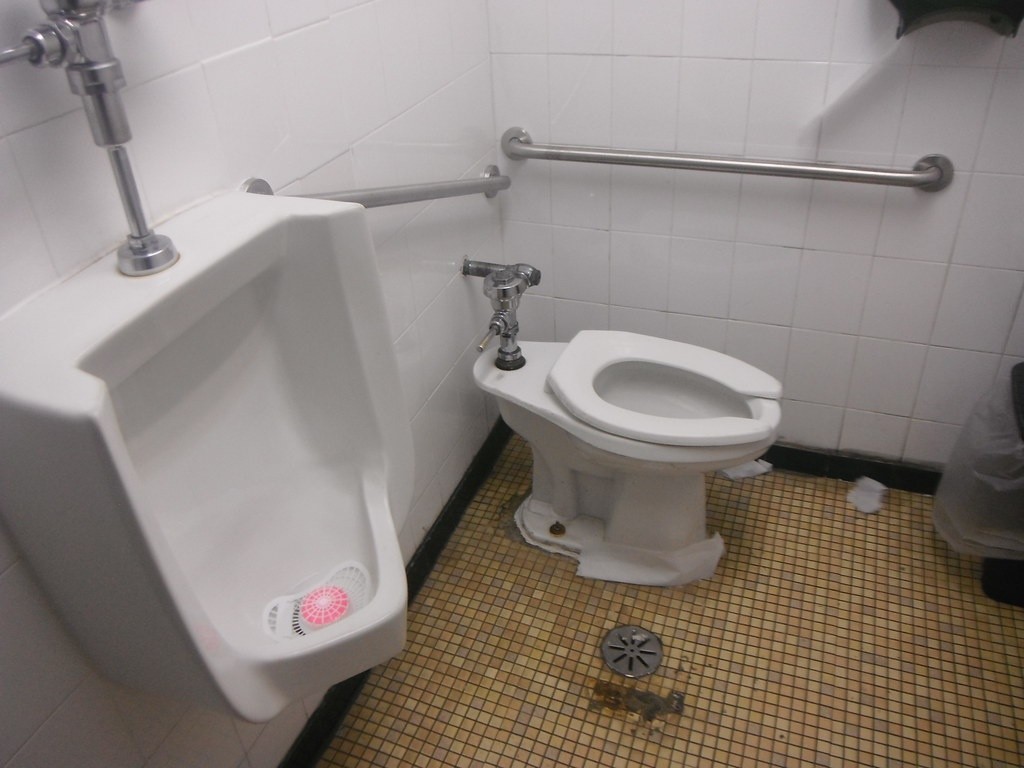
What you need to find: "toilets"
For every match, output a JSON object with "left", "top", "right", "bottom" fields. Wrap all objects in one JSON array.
[{"left": 471, "top": 328, "right": 785, "bottom": 589}]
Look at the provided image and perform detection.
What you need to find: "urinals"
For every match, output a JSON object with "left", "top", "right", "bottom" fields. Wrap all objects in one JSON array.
[{"left": 1, "top": 189, "right": 418, "bottom": 724}]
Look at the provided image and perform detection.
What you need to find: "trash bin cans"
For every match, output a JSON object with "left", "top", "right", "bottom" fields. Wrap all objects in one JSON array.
[{"left": 980, "top": 361, "right": 1024, "bottom": 608}]
